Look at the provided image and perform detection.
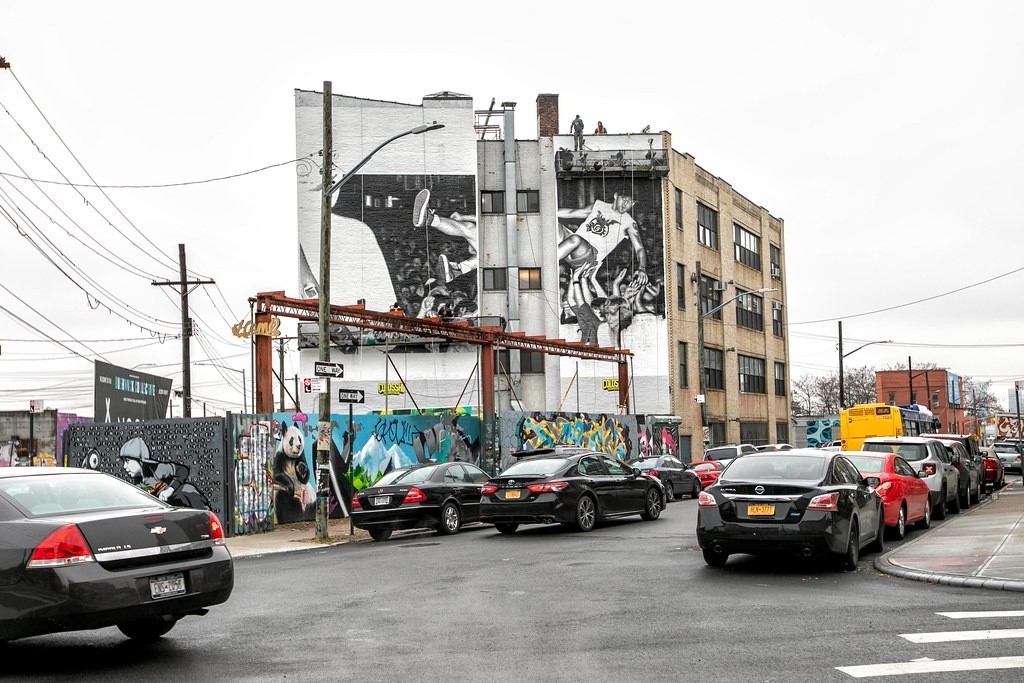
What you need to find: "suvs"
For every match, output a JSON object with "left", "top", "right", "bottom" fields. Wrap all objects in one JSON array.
[
  {"left": 703, "top": 443, "right": 759, "bottom": 466},
  {"left": 861, "top": 435, "right": 961, "bottom": 521}
]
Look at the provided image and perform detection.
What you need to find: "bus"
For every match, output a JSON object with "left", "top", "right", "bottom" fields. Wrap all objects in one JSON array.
[{"left": 839, "top": 403, "right": 942, "bottom": 448}]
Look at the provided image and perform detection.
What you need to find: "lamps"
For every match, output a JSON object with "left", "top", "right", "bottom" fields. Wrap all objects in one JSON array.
[
  {"left": 730, "top": 417, "right": 739, "bottom": 423},
  {"left": 727, "top": 347, "right": 736, "bottom": 351},
  {"left": 728, "top": 280, "right": 734, "bottom": 284}
]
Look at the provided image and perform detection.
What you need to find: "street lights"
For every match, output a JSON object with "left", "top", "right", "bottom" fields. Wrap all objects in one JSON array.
[
  {"left": 972, "top": 389, "right": 987, "bottom": 434},
  {"left": 315, "top": 79, "right": 445, "bottom": 539},
  {"left": 694, "top": 260, "right": 779, "bottom": 461},
  {"left": 951, "top": 379, "right": 967, "bottom": 436},
  {"left": 837, "top": 318, "right": 894, "bottom": 411},
  {"left": 908, "top": 355, "right": 934, "bottom": 406}
]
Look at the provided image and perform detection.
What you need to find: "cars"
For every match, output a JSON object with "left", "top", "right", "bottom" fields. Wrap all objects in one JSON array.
[
  {"left": 687, "top": 460, "right": 723, "bottom": 488},
  {"left": 696, "top": 449, "right": 886, "bottom": 572},
  {"left": 0, "top": 466, "right": 235, "bottom": 657},
  {"left": 1002, "top": 438, "right": 1024, "bottom": 455},
  {"left": 917, "top": 434, "right": 1005, "bottom": 509},
  {"left": 756, "top": 443, "right": 797, "bottom": 453},
  {"left": 831, "top": 450, "right": 934, "bottom": 541},
  {"left": 479, "top": 450, "right": 667, "bottom": 532},
  {"left": 349, "top": 461, "right": 493, "bottom": 542},
  {"left": 989, "top": 442, "right": 1023, "bottom": 476},
  {"left": 625, "top": 454, "right": 703, "bottom": 502}
]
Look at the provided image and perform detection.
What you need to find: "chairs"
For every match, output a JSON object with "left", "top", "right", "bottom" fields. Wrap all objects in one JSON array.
[
  {"left": 877, "top": 446, "right": 893, "bottom": 453},
  {"left": 751, "top": 462, "right": 775, "bottom": 477}
]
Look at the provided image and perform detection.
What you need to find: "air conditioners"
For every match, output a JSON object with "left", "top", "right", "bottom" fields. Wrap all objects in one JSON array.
[
  {"left": 772, "top": 301, "right": 781, "bottom": 310},
  {"left": 771, "top": 268, "right": 779, "bottom": 277},
  {"left": 713, "top": 281, "right": 727, "bottom": 291}
]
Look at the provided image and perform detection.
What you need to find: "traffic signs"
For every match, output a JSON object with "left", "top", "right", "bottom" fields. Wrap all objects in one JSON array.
[
  {"left": 337, "top": 387, "right": 367, "bottom": 406},
  {"left": 314, "top": 361, "right": 344, "bottom": 380}
]
